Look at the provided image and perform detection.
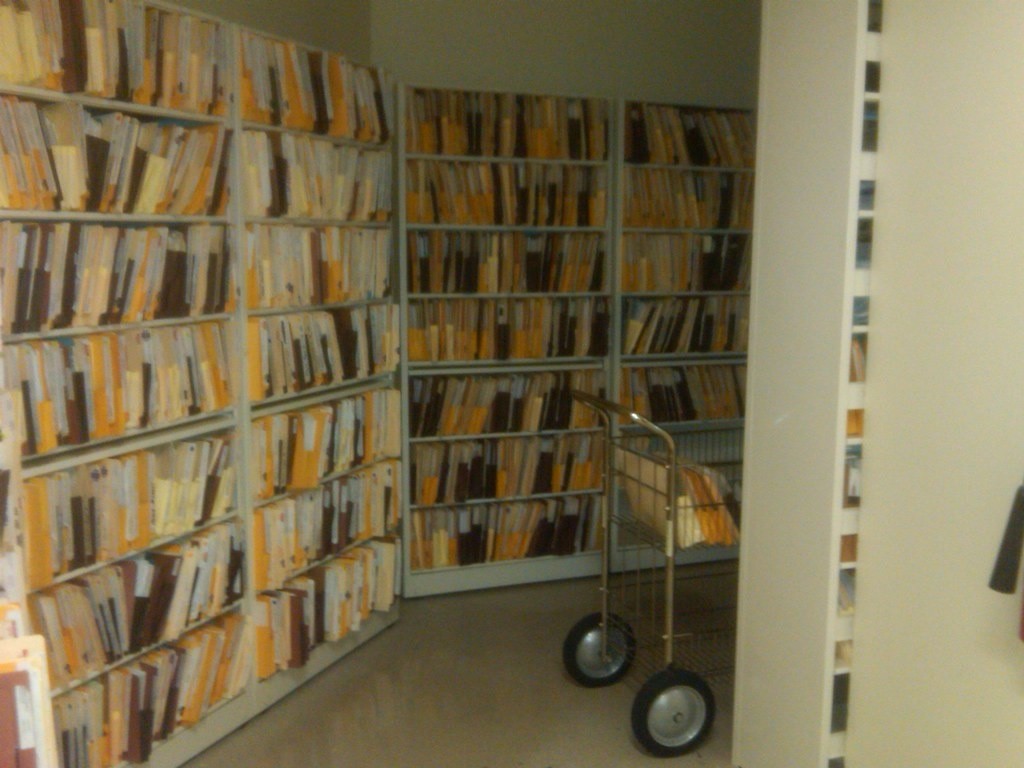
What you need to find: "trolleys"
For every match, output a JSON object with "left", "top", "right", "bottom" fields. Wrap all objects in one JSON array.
[{"left": 551, "top": 385, "right": 745, "bottom": 756}]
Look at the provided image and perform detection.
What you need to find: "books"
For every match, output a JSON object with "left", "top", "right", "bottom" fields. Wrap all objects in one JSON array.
[{"left": 0, "top": 0, "right": 868, "bottom": 767}]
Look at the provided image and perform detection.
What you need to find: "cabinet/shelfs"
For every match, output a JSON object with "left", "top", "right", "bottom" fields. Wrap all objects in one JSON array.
[
  {"left": 400, "top": 82, "right": 617, "bottom": 599},
  {"left": 610, "top": 90, "right": 758, "bottom": 576},
  {"left": 730, "top": 0, "right": 1024, "bottom": 768},
  {"left": 0, "top": 0, "right": 400, "bottom": 768}
]
